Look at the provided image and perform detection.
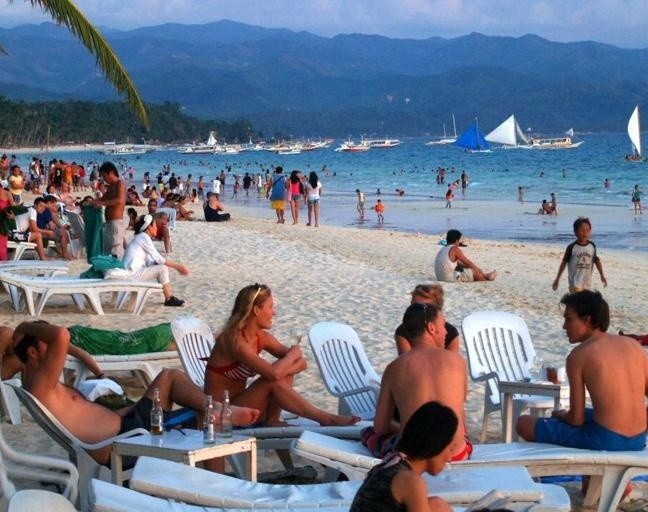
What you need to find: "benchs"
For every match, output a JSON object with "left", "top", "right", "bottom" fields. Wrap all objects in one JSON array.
[{"left": 290, "top": 431, "right": 648, "bottom": 512}]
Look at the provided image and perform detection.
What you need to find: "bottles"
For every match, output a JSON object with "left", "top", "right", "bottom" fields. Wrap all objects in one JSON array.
[
  {"left": 151, "top": 387, "right": 164, "bottom": 434},
  {"left": 203, "top": 395, "right": 215, "bottom": 444},
  {"left": 221, "top": 391, "right": 232, "bottom": 442}
]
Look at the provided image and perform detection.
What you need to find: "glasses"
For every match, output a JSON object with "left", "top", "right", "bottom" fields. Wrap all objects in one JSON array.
[{"left": 252, "top": 283, "right": 262, "bottom": 302}]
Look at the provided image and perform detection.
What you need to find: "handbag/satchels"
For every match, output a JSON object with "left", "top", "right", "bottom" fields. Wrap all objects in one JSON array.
[{"left": 287, "top": 188, "right": 292, "bottom": 201}]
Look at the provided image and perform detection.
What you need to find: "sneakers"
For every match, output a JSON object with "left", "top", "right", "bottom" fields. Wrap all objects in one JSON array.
[{"left": 165, "top": 296, "right": 184, "bottom": 306}]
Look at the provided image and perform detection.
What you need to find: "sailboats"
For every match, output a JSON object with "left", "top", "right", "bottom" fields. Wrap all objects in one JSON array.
[
  {"left": 624, "top": 104, "right": 646, "bottom": 162},
  {"left": 446, "top": 117, "right": 493, "bottom": 153},
  {"left": 485, "top": 115, "right": 533, "bottom": 150}
]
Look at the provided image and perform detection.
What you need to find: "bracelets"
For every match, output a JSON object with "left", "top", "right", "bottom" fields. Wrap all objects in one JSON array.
[{"left": 98, "top": 373, "right": 105, "bottom": 379}]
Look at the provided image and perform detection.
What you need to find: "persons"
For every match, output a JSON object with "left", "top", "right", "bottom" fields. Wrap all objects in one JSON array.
[
  {"left": 435, "top": 229, "right": 498, "bottom": 283},
  {"left": 515, "top": 289, "right": 646, "bottom": 504},
  {"left": 0, "top": 152, "right": 231, "bottom": 306},
  {"left": 231, "top": 162, "right": 272, "bottom": 199},
  {"left": 633, "top": 185, "right": 644, "bottom": 214},
  {"left": 349, "top": 403, "right": 457, "bottom": 511},
  {"left": 358, "top": 303, "right": 472, "bottom": 463},
  {"left": 551, "top": 217, "right": 607, "bottom": 291},
  {"left": 1, "top": 321, "right": 28, "bottom": 383},
  {"left": 63, "top": 324, "right": 221, "bottom": 381},
  {"left": 394, "top": 285, "right": 459, "bottom": 423},
  {"left": 605, "top": 179, "right": 609, "bottom": 188},
  {"left": 431, "top": 165, "right": 467, "bottom": 208},
  {"left": 519, "top": 187, "right": 524, "bottom": 204},
  {"left": 4, "top": 320, "right": 261, "bottom": 474},
  {"left": 204, "top": 282, "right": 360, "bottom": 426},
  {"left": 376, "top": 188, "right": 405, "bottom": 223},
  {"left": 356, "top": 189, "right": 365, "bottom": 218},
  {"left": 266, "top": 164, "right": 328, "bottom": 225},
  {"left": 542, "top": 193, "right": 558, "bottom": 215},
  {"left": 627, "top": 153, "right": 640, "bottom": 160}
]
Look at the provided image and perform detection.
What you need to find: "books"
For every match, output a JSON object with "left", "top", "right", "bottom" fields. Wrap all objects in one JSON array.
[{"left": 463, "top": 489, "right": 514, "bottom": 512}]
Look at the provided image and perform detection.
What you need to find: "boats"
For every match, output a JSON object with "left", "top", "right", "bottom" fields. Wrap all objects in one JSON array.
[
  {"left": 86, "top": 137, "right": 155, "bottom": 155},
  {"left": 334, "top": 137, "right": 371, "bottom": 152},
  {"left": 518, "top": 138, "right": 584, "bottom": 150},
  {"left": 177, "top": 133, "right": 335, "bottom": 153},
  {"left": 360, "top": 137, "right": 402, "bottom": 147}
]
